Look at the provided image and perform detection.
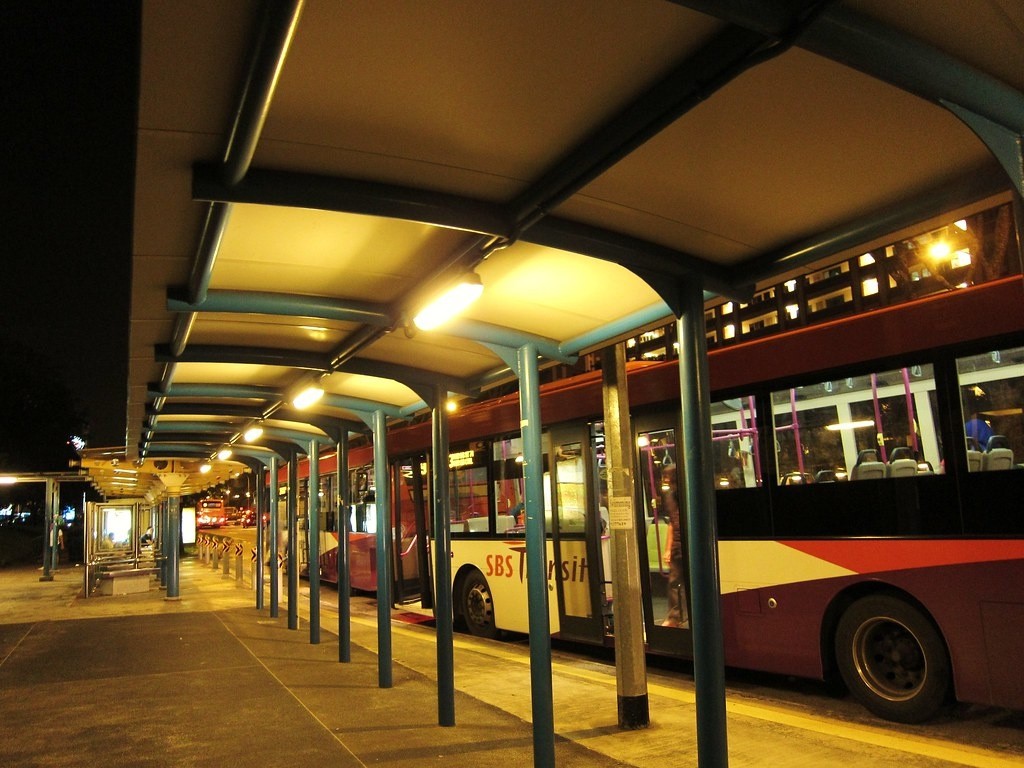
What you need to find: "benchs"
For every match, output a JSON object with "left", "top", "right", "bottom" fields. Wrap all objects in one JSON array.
[{"left": 95, "top": 547, "right": 162, "bottom": 596}]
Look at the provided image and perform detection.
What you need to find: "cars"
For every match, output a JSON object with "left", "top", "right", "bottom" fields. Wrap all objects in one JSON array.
[{"left": 225, "top": 507, "right": 257, "bottom": 526}]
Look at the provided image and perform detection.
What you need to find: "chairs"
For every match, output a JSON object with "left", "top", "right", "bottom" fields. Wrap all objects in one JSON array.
[
  {"left": 887, "top": 447, "right": 934, "bottom": 478},
  {"left": 850, "top": 449, "right": 886, "bottom": 480},
  {"left": 940, "top": 437, "right": 984, "bottom": 474},
  {"left": 982, "top": 434, "right": 1014, "bottom": 470},
  {"left": 646, "top": 516, "right": 670, "bottom": 572}
]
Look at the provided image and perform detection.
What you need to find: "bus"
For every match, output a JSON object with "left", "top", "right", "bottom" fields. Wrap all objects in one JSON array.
[
  {"left": 266, "top": 444, "right": 516, "bottom": 592},
  {"left": 386, "top": 271, "right": 1024, "bottom": 723},
  {"left": 197, "top": 499, "right": 226, "bottom": 530}
]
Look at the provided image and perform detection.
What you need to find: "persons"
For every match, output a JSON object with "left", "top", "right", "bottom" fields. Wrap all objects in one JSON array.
[
  {"left": 140, "top": 526, "right": 153, "bottom": 546},
  {"left": 660, "top": 461, "right": 689, "bottom": 627},
  {"left": 100, "top": 532, "right": 120, "bottom": 549},
  {"left": 965, "top": 396, "right": 996, "bottom": 454},
  {"left": 49, "top": 522, "right": 64, "bottom": 566}
]
